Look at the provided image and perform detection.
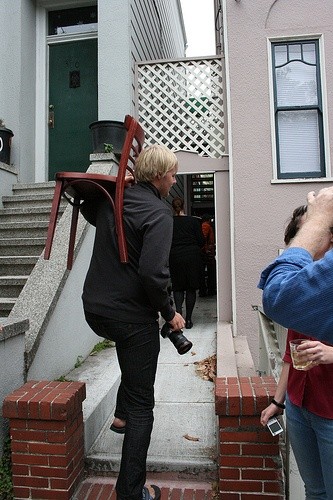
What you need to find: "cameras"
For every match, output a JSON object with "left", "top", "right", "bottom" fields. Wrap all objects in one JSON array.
[
  {"left": 161, "top": 321, "right": 193, "bottom": 355},
  {"left": 266, "top": 417, "right": 283, "bottom": 436}
]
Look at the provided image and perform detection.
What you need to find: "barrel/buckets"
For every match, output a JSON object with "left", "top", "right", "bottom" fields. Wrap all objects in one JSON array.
[
  {"left": 0, "top": 127, "right": 14, "bottom": 165},
  {"left": 89, "top": 121, "right": 126, "bottom": 162}
]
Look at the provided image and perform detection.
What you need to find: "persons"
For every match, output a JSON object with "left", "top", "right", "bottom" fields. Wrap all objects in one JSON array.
[
  {"left": 169, "top": 199, "right": 206, "bottom": 330},
  {"left": 199, "top": 213, "right": 216, "bottom": 297},
  {"left": 79, "top": 143, "right": 185, "bottom": 500},
  {"left": 256, "top": 187, "right": 331, "bottom": 346},
  {"left": 259, "top": 207, "right": 333, "bottom": 500}
]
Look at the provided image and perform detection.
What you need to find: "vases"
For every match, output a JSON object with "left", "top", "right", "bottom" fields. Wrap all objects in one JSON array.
[{"left": 89, "top": 120, "right": 128, "bottom": 154}]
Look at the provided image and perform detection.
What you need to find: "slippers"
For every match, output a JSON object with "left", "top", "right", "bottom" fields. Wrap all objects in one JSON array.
[
  {"left": 109, "top": 423, "right": 126, "bottom": 434},
  {"left": 141, "top": 484, "right": 161, "bottom": 500}
]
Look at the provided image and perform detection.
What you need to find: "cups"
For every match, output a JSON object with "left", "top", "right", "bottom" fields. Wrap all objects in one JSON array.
[{"left": 290, "top": 339, "right": 313, "bottom": 370}]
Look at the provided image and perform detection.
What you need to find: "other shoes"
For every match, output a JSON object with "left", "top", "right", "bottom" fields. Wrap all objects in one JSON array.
[{"left": 185, "top": 321, "right": 193, "bottom": 329}]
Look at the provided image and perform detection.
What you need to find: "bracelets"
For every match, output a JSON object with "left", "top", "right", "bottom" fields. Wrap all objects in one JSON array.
[{"left": 271, "top": 399, "right": 286, "bottom": 409}]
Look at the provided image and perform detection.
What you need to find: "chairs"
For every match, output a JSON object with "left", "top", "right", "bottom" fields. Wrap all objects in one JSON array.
[{"left": 44, "top": 114, "right": 145, "bottom": 270}]
